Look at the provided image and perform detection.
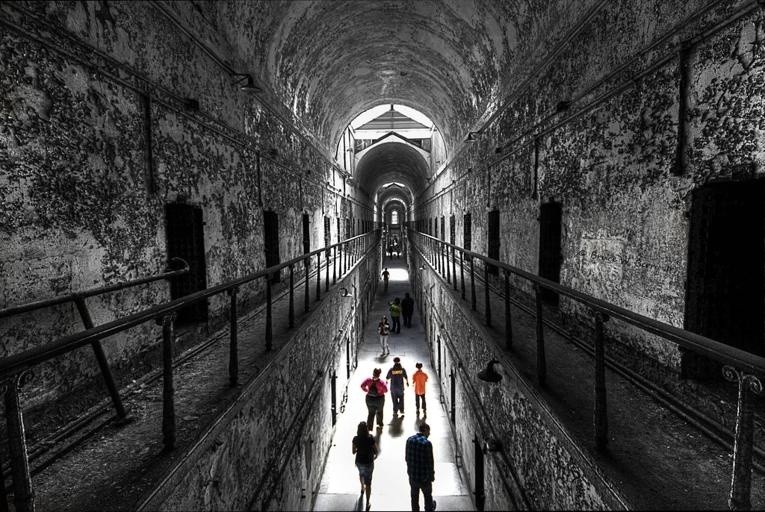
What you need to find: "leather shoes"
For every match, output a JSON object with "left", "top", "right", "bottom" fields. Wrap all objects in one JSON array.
[
  {"left": 366, "top": 505, "right": 370, "bottom": 511},
  {"left": 430, "top": 500, "right": 436, "bottom": 511}
]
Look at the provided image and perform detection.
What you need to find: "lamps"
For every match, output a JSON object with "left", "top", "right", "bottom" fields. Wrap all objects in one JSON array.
[{"left": 474, "top": 357, "right": 503, "bottom": 385}]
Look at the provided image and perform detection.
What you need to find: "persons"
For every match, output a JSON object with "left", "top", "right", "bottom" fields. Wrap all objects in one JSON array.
[
  {"left": 352, "top": 421, "right": 377, "bottom": 512},
  {"left": 401, "top": 292, "right": 414, "bottom": 328},
  {"left": 385, "top": 357, "right": 409, "bottom": 416},
  {"left": 388, "top": 239, "right": 401, "bottom": 259},
  {"left": 388, "top": 297, "right": 403, "bottom": 334},
  {"left": 361, "top": 367, "right": 387, "bottom": 432},
  {"left": 382, "top": 267, "right": 389, "bottom": 288},
  {"left": 412, "top": 363, "right": 429, "bottom": 419},
  {"left": 405, "top": 423, "right": 436, "bottom": 511},
  {"left": 376, "top": 315, "right": 390, "bottom": 356}
]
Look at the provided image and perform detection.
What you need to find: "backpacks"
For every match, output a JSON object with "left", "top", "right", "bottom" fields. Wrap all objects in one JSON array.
[{"left": 367, "top": 378, "right": 379, "bottom": 397}]
